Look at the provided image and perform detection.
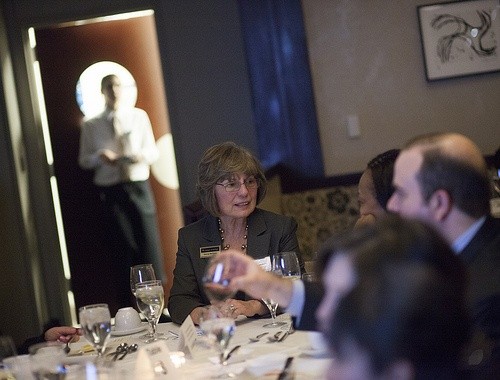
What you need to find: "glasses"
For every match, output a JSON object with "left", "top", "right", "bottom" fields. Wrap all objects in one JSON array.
[{"left": 215, "top": 178, "right": 260, "bottom": 192}]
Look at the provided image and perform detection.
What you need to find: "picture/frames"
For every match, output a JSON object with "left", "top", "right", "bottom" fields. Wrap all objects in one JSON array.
[{"left": 417, "top": 0, "right": 500, "bottom": 82}]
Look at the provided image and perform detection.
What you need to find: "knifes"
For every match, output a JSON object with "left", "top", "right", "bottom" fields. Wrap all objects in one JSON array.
[{"left": 277, "top": 356, "right": 293, "bottom": 380}]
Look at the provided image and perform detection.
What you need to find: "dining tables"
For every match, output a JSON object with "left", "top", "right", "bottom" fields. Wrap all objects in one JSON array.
[{"left": 57, "top": 322, "right": 323, "bottom": 379}]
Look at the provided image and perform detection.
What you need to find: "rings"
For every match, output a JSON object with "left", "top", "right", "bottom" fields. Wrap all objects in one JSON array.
[{"left": 229, "top": 305, "right": 236, "bottom": 312}]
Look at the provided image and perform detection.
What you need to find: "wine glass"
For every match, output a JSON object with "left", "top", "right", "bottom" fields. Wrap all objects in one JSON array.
[
  {"left": 202, "top": 316, "right": 235, "bottom": 367},
  {"left": 135, "top": 279, "right": 166, "bottom": 345},
  {"left": 259, "top": 269, "right": 287, "bottom": 328},
  {"left": 78, "top": 303, "right": 112, "bottom": 362},
  {"left": 129, "top": 264, "right": 163, "bottom": 340}
]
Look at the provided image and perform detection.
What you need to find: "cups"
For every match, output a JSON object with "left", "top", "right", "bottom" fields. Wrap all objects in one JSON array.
[
  {"left": 273, "top": 250, "right": 302, "bottom": 280},
  {"left": 3, "top": 354, "right": 37, "bottom": 380},
  {"left": 302, "top": 272, "right": 320, "bottom": 283},
  {"left": 114, "top": 306, "right": 142, "bottom": 332},
  {"left": 29, "top": 342, "right": 66, "bottom": 380}
]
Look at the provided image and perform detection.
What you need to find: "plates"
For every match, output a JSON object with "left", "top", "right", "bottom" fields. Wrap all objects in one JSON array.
[{"left": 110, "top": 324, "right": 147, "bottom": 336}]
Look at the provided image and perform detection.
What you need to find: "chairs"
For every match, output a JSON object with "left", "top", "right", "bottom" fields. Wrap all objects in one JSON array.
[{"left": 283, "top": 183, "right": 361, "bottom": 259}]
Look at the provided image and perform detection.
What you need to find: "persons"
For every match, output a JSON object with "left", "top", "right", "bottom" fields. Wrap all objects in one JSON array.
[
  {"left": 167, "top": 143, "right": 305, "bottom": 327},
  {"left": 352, "top": 149, "right": 401, "bottom": 227},
  {"left": 78, "top": 74, "right": 165, "bottom": 302},
  {"left": 208, "top": 130, "right": 500, "bottom": 380},
  {"left": 315, "top": 220, "right": 470, "bottom": 380},
  {"left": 0, "top": 325, "right": 85, "bottom": 367}
]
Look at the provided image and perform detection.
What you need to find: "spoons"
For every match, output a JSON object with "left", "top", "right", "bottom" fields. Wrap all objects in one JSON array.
[
  {"left": 106, "top": 342, "right": 138, "bottom": 361},
  {"left": 64, "top": 331, "right": 78, "bottom": 353}
]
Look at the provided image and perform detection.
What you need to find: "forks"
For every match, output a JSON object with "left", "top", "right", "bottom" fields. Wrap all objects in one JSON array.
[
  {"left": 267, "top": 330, "right": 283, "bottom": 342},
  {"left": 249, "top": 332, "right": 268, "bottom": 342}
]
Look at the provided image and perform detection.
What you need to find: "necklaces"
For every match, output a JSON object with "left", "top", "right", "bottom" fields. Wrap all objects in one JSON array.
[{"left": 216, "top": 216, "right": 248, "bottom": 258}]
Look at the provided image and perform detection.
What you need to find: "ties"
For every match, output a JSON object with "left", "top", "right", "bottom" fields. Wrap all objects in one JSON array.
[{"left": 110, "top": 116, "right": 119, "bottom": 139}]
